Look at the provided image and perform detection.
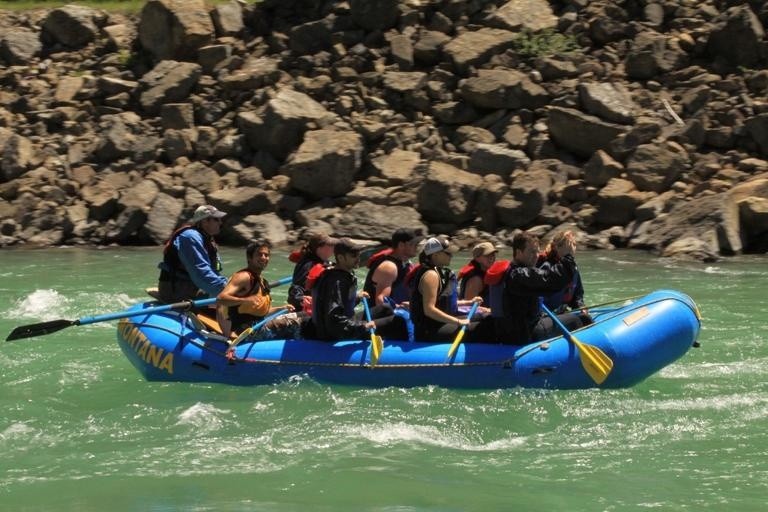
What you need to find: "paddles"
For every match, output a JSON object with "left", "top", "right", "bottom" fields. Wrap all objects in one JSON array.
[
  {"left": 227, "top": 309, "right": 290, "bottom": 352},
  {"left": 447, "top": 303, "right": 477, "bottom": 359},
  {"left": 538, "top": 298, "right": 614, "bottom": 384},
  {"left": 362, "top": 299, "right": 383, "bottom": 364},
  {"left": 6, "top": 277, "right": 293, "bottom": 340}
]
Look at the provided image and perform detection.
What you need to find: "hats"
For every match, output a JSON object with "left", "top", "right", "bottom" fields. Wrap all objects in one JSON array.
[
  {"left": 311, "top": 233, "right": 340, "bottom": 246},
  {"left": 334, "top": 238, "right": 367, "bottom": 254},
  {"left": 392, "top": 228, "right": 425, "bottom": 245},
  {"left": 472, "top": 242, "right": 499, "bottom": 257},
  {"left": 423, "top": 237, "right": 459, "bottom": 256},
  {"left": 193, "top": 205, "right": 227, "bottom": 223}
]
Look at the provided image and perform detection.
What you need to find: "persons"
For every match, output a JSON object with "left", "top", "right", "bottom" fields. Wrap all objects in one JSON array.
[
  {"left": 407, "top": 236, "right": 495, "bottom": 343},
  {"left": 458, "top": 240, "right": 499, "bottom": 305},
  {"left": 286, "top": 232, "right": 338, "bottom": 316},
  {"left": 360, "top": 228, "right": 425, "bottom": 340},
  {"left": 499, "top": 231, "right": 596, "bottom": 343},
  {"left": 309, "top": 237, "right": 408, "bottom": 341},
  {"left": 536, "top": 229, "right": 588, "bottom": 314},
  {"left": 157, "top": 205, "right": 231, "bottom": 310},
  {"left": 215, "top": 238, "right": 301, "bottom": 340}
]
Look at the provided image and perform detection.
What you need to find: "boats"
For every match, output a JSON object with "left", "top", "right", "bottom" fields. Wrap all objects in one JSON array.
[{"left": 115, "top": 286, "right": 706, "bottom": 390}]
